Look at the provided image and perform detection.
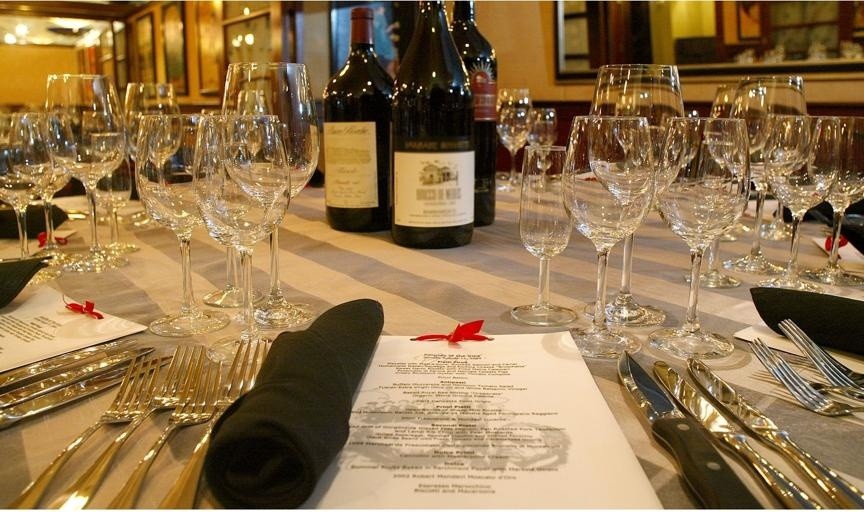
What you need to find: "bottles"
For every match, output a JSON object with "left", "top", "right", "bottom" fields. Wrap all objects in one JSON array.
[
  {"left": 447, "top": 1, "right": 497, "bottom": 226},
  {"left": 320, "top": 6, "right": 396, "bottom": 231},
  {"left": 372, "top": 1, "right": 476, "bottom": 245}
]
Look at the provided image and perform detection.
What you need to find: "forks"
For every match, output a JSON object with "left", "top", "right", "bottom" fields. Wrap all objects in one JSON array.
[
  {"left": 773, "top": 317, "right": 864, "bottom": 402},
  {"left": 157, "top": 336, "right": 270, "bottom": 510},
  {"left": 48, "top": 346, "right": 209, "bottom": 510},
  {"left": 3, "top": 355, "right": 160, "bottom": 508},
  {"left": 108, "top": 360, "right": 225, "bottom": 510},
  {"left": 745, "top": 336, "right": 863, "bottom": 416}
]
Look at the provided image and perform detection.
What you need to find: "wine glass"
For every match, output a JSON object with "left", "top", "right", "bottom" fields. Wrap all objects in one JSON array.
[
  {"left": 134, "top": 112, "right": 232, "bottom": 337},
  {"left": 134, "top": 98, "right": 182, "bottom": 230},
  {"left": 121, "top": 81, "right": 175, "bottom": 220},
  {"left": 500, "top": 108, "right": 534, "bottom": 193},
  {"left": 91, "top": 132, "right": 140, "bottom": 254},
  {"left": 526, "top": 107, "right": 558, "bottom": 204},
  {"left": 509, "top": 143, "right": 580, "bottom": 329},
  {"left": 646, "top": 118, "right": 752, "bottom": 358},
  {"left": 803, "top": 115, "right": 864, "bottom": 292},
  {"left": 81, "top": 111, "right": 127, "bottom": 224},
  {"left": 559, "top": 114, "right": 655, "bottom": 360},
  {"left": 500, "top": 86, "right": 531, "bottom": 185},
  {"left": 193, "top": 108, "right": 267, "bottom": 309},
  {"left": 682, "top": 139, "right": 746, "bottom": 289},
  {"left": 8, "top": 110, "right": 82, "bottom": 267},
  {"left": 46, "top": 72, "right": 130, "bottom": 273},
  {"left": 722, "top": 75, "right": 811, "bottom": 276},
  {"left": 752, "top": 114, "right": 845, "bottom": 294},
  {"left": 3, "top": 114, "right": 62, "bottom": 286},
  {"left": 219, "top": 62, "right": 321, "bottom": 331},
  {"left": 703, "top": 86, "right": 751, "bottom": 240},
  {"left": 191, "top": 112, "right": 292, "bottom": 366},
  {"left": 582, "top": 64, "right": 688, "bottom": 330}
]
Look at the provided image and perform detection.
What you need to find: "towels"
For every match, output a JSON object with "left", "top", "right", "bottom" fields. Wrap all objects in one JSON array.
[
  {"left": 1, "top": 165, "right": 91, "bottom": 311},
  {"left": 745, "top": 195, "right": 864, "bottom": 361}
]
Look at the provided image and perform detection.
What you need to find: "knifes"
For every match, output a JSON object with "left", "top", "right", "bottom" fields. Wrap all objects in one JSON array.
[
  {"left": 617, "top": 350, "right": 765, "bottom": 509},
  {"left": 652, "top": 358, "right": 825, "bottom": 510},
  {"left": 683, "top": 355, "right": 864, "bottom": 510}
]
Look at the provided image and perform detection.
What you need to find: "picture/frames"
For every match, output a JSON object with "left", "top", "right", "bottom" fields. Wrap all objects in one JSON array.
[{"left": 74, "top": 2, "right": 275, "bottom": 98}]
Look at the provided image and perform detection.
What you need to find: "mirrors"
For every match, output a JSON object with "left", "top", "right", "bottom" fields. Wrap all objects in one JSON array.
[{"left": 554, "top": 1, "right": 864, "bottom": 85}]
